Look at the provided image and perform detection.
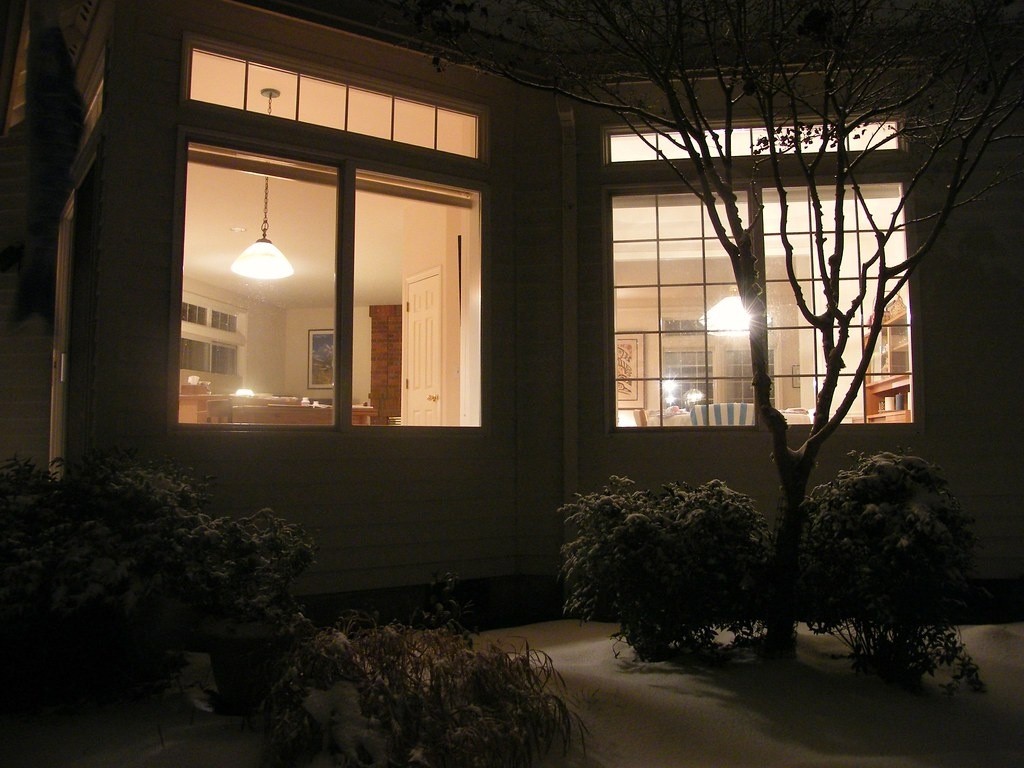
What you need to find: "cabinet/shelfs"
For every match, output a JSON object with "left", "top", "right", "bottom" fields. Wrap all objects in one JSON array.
[{"left": 861, "top": 309, "right": 910, "bottom": 423}]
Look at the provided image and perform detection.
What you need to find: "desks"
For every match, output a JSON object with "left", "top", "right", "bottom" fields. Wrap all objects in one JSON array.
[
  {"left": 653, "top": 413, "right": 811, "bottom": 425},
  {"left": 232, "top": 403, "right": 380, "bottom": 425}
]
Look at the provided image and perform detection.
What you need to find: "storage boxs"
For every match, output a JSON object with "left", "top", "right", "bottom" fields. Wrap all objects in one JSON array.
[{"left": 867, "top": 311, "right": 891, "bottom": 329}]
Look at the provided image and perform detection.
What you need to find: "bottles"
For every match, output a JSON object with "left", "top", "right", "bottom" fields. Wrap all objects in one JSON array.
[
  {"left": 312, "top": 401, "right": 319, "bottom": 406},
  {"left": 301, "top": 397, "right": 310, "bottom": 405}
]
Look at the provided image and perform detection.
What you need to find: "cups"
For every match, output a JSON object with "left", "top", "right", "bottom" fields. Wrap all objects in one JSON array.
[
  {"left": 896, "top": 393, "right": 904, "bottom": 410},
  {"left": 188, "top": 376, "right": 200, "bottom": 385},
  {"left": 885, "top": 397, "right": 894, "bottom": 411},
  {"left": 879, "top": 402, "right": 885, "bottom": 411}
]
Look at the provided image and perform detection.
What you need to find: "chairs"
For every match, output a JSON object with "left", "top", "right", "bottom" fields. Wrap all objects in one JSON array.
[
  {"left": 689, "top": 403, "right": 757, "bottom": 426},
  {"left": 633, "top": 410, "right": 647, "bottom": 426},
  {"left": 206, "top": 398, "right": 233, "bottom": 423}
]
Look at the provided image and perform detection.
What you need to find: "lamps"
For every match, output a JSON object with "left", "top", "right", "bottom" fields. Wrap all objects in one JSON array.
[
  {"left": 229, "top": 87, "right": 296, "bottom": 281},
  {"left": 698, "top": 285, "right": 773, "bottom": 338},
  {"left": 680, "top": 384, "right": 706, "bottom": 403}
]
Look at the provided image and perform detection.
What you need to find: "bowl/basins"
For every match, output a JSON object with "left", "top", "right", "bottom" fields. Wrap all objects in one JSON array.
[{"left": 198, "top": 381, "right": 211, "bottom": 385}]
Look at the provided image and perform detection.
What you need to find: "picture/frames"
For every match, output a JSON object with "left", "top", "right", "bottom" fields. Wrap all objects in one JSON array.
[
  {"left": 791, "top": 365, "right": 800, "bottom": 388},
  {"left": 307, "top": 329, "right": 337, "bottom": 390},
  {"left": 616, "top": 333, "right": 645, "bottom": 410}
]
